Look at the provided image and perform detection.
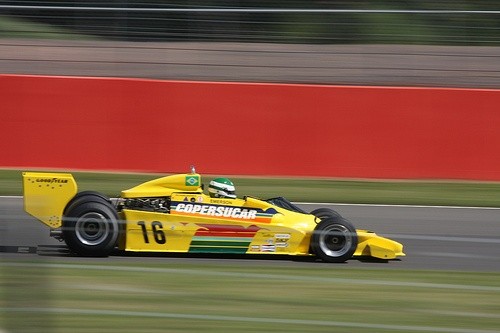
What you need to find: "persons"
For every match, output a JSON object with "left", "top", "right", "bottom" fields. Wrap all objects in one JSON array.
[{"left": 209, "top": 176, "right": 236, "bottom": 199}]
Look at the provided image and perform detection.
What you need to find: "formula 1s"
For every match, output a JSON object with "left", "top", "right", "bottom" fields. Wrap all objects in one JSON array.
[{"left": 23, "top": 166, "right": 406, "bottom": 262}]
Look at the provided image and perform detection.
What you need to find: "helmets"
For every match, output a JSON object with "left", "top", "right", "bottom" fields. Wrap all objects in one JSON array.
[{"left": 208, "top": 177, "right": 237, "bottom": 199}]
